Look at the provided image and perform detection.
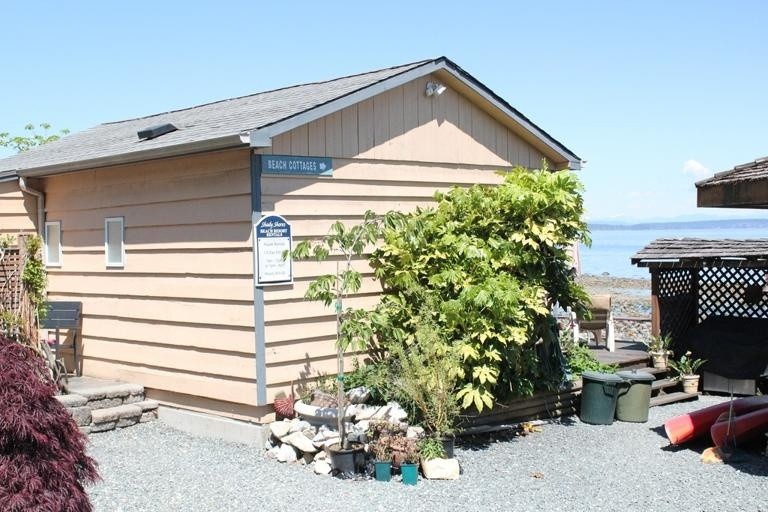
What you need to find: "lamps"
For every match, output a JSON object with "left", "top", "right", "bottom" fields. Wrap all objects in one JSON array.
[{"left": 427, "top": 82, "right": 447, "bottom": 98}]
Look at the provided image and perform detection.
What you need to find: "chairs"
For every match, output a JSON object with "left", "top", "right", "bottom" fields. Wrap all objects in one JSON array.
[
  {"left": 35, "top": 301, "right": 82, "bottom": 382},
  {"left": 571, "top": 294, "right": 615, "bottom": 352}
]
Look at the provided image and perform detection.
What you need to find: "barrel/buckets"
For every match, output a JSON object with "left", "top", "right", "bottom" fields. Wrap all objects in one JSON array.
[
  {"left": 579, "top": 368, "right": 623, "bottom": 426},
  {"left": 614, "top": 369, "right": 658, "bottom": 423}
]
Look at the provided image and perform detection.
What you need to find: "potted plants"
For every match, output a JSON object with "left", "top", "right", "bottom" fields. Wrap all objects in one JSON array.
[
  {"left": 282, "top": 209, "right": 454, "bottom": 483},
  {"left": 666, "top": 351, "right": 709, "bottom": 393},
  {"left": 640, "top": 331, "right": 674, "bottom": 368}
]
